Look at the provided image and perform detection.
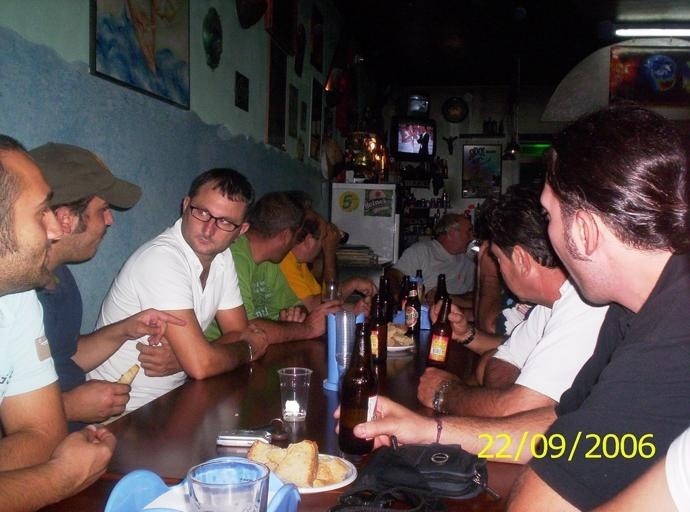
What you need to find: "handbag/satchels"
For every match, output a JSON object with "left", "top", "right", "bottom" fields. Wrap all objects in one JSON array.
[{"left": 340, "top": 443, "right": 501, "bottom": 501}]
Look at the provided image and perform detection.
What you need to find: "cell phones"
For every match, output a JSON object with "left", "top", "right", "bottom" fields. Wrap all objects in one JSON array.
[{"left": 216, "top": 428, "right": 272, "bottom": 447}]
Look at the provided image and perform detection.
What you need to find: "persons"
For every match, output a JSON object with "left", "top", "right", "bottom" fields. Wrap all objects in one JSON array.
[
  {"left": 28, "top": 142, "right": 188, "bottom": 434},
  {"left": 332, "top": 102, "right": 690, "bottom": 512},
  {"left": 309, "top": 224, "right": 337, "bottom": 286},
  {"left": 0, "top": 134, "right": 119, "bottom": 512},
  {"left": 85, "top": 167, "right": 270, "bottom": 425},
  {"left": 203, "top": 190, "right": 344, "bottom": 347},
  {"left": 279, "top": 207, "right": 379, "bottom": 318}
]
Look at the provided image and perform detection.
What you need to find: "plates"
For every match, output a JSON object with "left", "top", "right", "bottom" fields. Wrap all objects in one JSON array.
[{"left": 298, "top": 453, "right": 359, "bottom": 494}]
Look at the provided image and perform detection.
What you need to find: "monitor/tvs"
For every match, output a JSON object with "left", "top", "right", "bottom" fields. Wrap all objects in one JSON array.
[{"left": 391, "top": 114, "right": 436, "bottom": 161}]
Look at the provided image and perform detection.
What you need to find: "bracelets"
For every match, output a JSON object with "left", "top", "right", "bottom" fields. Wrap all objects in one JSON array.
[
  {"left": 248, "top": 366, "right": 255, "bottom": 380},
  {"left": 248, "top": 343, "right": 254, "bottom": 363}
]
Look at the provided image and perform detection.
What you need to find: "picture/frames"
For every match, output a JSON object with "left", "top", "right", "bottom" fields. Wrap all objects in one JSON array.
[
  {"left": 88, "top": 0, "right": 191, "bottom": 109},
  {"left": 265, "top": 38, "right": 287, "bottom": 152}
]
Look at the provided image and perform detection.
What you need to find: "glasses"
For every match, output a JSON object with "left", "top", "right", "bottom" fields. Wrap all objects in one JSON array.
[{"left": 190, "top": 202, "right": 241, "bottom": 231}]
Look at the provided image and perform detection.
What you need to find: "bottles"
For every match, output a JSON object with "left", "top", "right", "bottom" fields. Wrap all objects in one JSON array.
[
  {"left": 398, "top": 155, "right": 450, "bottom": 236},
  {"left": 339, "top": 268, "right": 453, "bottom": 456},
  {"left": 465, "top": 202, "right": 482, "bottom": 233}
]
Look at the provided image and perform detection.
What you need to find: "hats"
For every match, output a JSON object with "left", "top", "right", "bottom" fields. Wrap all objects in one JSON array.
[{"left": 28, "top": 142, "right": 141, "bottom": 212}]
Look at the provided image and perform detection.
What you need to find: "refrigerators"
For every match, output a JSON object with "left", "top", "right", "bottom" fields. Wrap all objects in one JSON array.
[{"left": 331, "top": 182, "right": 400, "bottom": 265}]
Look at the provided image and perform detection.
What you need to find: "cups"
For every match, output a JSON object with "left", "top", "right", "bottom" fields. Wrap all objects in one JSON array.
[
  {"left": 321, "top": 277, "right": 343, "bottom": 304},
  {"left": 277, "top": 366, "right": 313, "bottom": 422},
  {"left": 187, "top": 457, "right": 270, "bottom": 512}
]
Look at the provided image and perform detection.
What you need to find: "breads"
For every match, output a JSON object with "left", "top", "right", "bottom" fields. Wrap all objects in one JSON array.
[
  {"left": 247, "top": 438, "right": 353, "bottom": 491},
  {"left": 387, "top": 322, "right": 415, "bottom": 347},
  {"left": 116, "top": 363, "right": 140, "bottom": 385}
]
